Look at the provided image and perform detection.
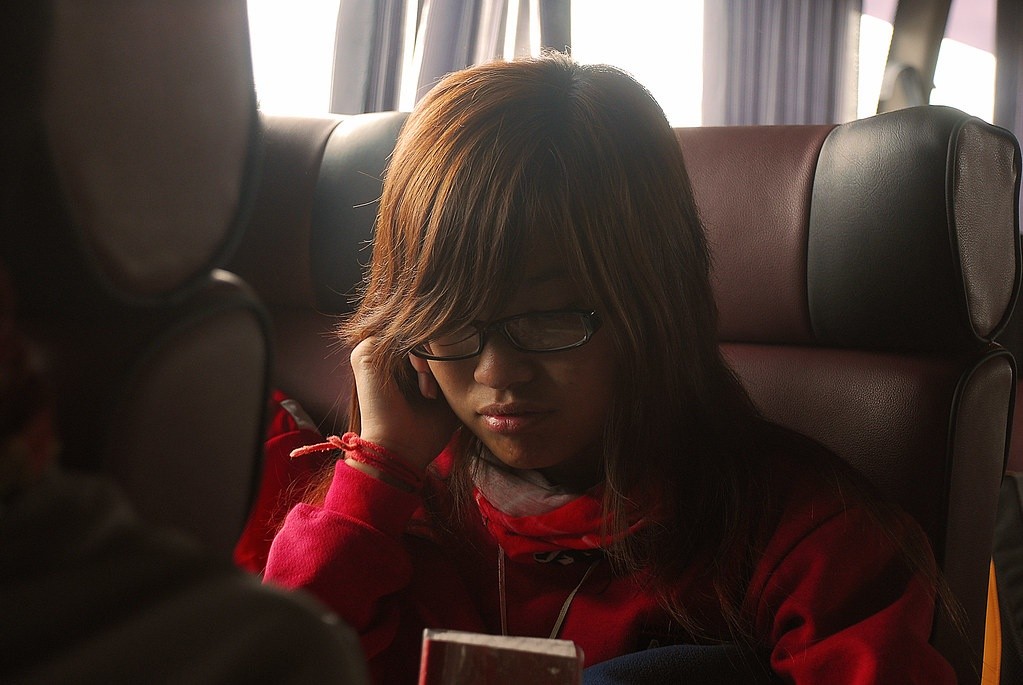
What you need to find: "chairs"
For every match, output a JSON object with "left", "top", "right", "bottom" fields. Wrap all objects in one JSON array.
[
  {"left": 0, "top": 1, "right": 368, "bottom": 685},
  {"left": 228, "top": 103, "right": 1023, "bottom": 685}
]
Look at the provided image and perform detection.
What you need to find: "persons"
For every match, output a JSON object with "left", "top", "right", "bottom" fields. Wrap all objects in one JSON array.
[
  {"left": 0, "top": 324, "right": 368, "bottom": 685},
  {"left": 262, "top": 62, "right": 958, "bottom": 685}
]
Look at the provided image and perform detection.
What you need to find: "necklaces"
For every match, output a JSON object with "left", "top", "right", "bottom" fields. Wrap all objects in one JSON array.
[{"left": 498, "top": 543, "right": 602, "bottom": 637}]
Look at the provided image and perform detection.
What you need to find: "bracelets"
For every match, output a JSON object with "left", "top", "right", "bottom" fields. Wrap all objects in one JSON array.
[{"left": 290, "top": 432, "right": 429, "bottom": 490}]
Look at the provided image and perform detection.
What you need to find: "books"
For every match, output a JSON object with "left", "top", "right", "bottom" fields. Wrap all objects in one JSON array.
[{"left": 419, "top": 627, "right": 585, "bottom": 685}]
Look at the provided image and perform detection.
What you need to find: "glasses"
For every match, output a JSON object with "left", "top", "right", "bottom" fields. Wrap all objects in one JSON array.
[{"left": 403, "top": 291, "right": 605, "bottom": 361}]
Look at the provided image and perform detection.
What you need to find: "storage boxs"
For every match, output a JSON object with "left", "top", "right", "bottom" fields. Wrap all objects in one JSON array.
[{"left": 419, "top": 627, "right": 584, "bottom": 685}]
